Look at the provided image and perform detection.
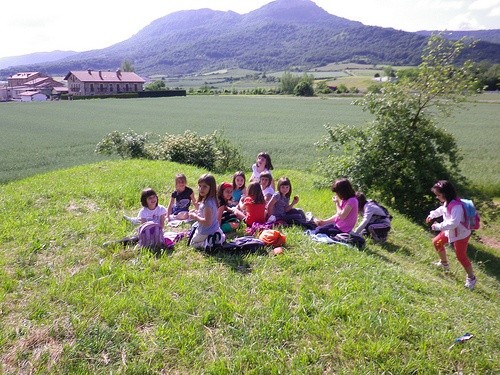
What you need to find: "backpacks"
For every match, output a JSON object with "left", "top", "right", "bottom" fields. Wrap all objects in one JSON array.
[
  {"left": 135, "top": 221, "right": 164, "bottom": 250},
  {"left": 447, "top": 198, "right": 480, "bottom": 230},
  {"left": 221, "top": 237, "right": 265, "bottom": 252},
  {"left": 365, "top": 198, "right": 393, "bottom": 221}
]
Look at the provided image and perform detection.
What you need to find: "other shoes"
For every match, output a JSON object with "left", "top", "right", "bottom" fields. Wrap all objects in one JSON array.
[
  {"left": 214, "top": 232, "right": 221, "bottom": 249},
  {"left": 123, "top": 215, "right": 138, "bottom": 226},
  {"left": 205, "top": 235, "right": 215, "bottom": 254}
]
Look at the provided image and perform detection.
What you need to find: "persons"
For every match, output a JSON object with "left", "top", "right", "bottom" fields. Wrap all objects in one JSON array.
[
  {"left": 426, "top": 181, "right": 477, "bottom": 291},
  {"left": 260, "top": 171, "right": 274, "bottom": 204},
  {"left": 230, "top": 171, "right": 247, "bottom": 221},
  {"left": 315, "top": 178, "right": 358, "bottom": 236},
  {"left": 234, "top": 182, "right": 266, "bottom": 227},
  {"left": 357, "top": 194, "right": 391, "bottom": 243},
  {"left": 267, "top": 177, "right": 306, "bottom": 226},
  {"left": 353, "top": 185, "right": 360, "bottom": 197},
  {"left": 188, "top": 174, "right": 221, "bottom": 254},
  {"left": 167, "top": 174, "right": 199, "bottom": 222},
  {"left": 138, "top": 188, "right": 167, "bottom": 230},
  {"left": 248, "top": 153, "right": 275, "bottom": 192},
  {"left": 218, "top": 183, "right": 237, "bottom": 233}
]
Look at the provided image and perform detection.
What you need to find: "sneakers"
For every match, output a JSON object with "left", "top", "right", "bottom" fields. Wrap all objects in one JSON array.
[
  {"left": 435, "top": 261, "right": 450, "bottom": 270},
  {"left": 465, "top": 275, "right": 476, "bottom": 289}
]
